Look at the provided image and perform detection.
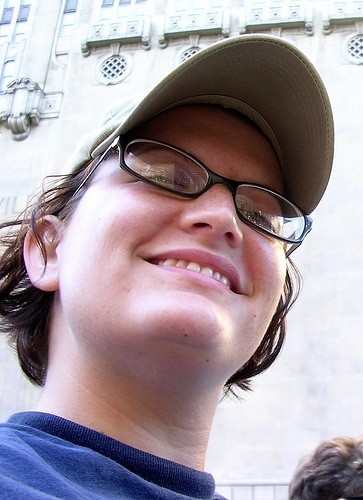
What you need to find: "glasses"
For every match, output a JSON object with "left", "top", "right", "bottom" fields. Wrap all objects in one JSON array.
[{"left": 54, "top": 134, "right": 313, "bottom": 260}]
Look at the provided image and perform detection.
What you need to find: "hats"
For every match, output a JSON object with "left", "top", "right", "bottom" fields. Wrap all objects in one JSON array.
[{"left": 47, "top": 32, "right": 334, "bottom": 217}]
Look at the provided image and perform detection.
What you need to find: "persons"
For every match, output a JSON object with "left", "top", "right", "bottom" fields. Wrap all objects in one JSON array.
[
  {"left": 289, "top": 436, "right": 363, "bottom": 500},
  {"left": 0, "top": 35, "right": 336, "bottom": 500}
]
